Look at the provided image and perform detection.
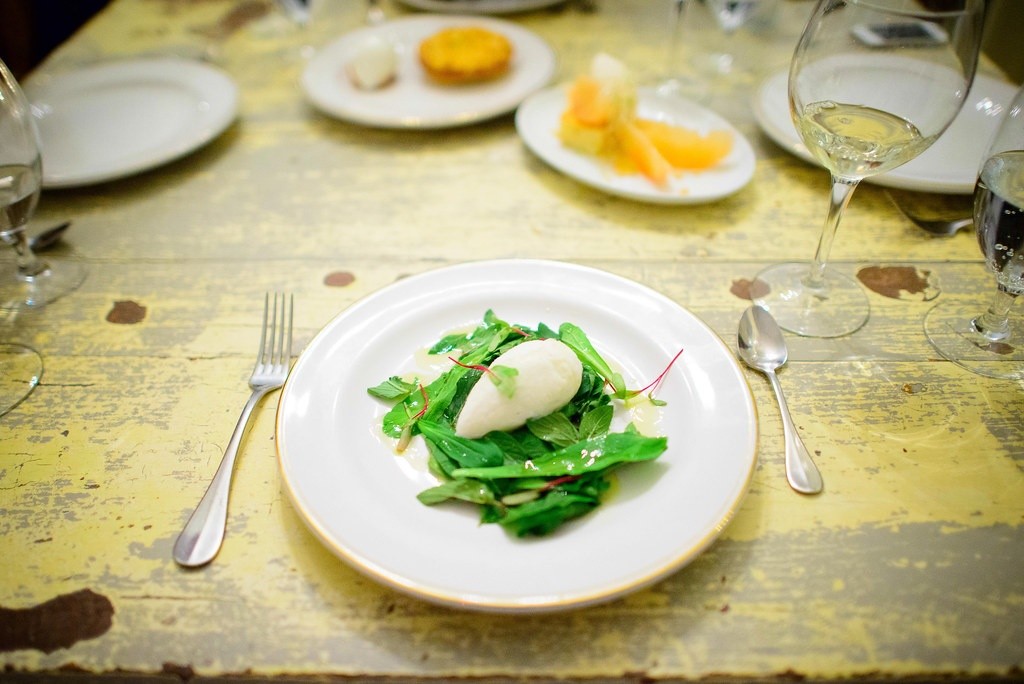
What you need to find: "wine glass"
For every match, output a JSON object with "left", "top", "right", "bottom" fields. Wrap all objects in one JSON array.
[
  {"left": 923, "top": 81, "right": 1024, "bottom": 382},
  {"left": 0, "top": 61, "right": 90, "bottom": 312},
  {"left": 748, "top": 0, "right": 990, "bottom": 339}
]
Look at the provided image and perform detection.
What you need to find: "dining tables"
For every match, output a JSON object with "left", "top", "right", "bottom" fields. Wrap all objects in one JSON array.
[{"left": 0, "top": 0, "right": 1024, "bottom": 684}]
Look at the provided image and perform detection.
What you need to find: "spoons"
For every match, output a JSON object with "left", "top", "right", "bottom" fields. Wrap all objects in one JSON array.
[
  {"left": 20, "top": 220, "right": 72, "bottom": 250},
  {"left": 738, "top": 304, "right": 823, "bottom": 496}
]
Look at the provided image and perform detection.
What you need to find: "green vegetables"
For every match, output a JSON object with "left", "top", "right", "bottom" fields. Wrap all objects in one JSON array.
[{"left": 367, "top": 308, "right": 683, "bottom": 541}]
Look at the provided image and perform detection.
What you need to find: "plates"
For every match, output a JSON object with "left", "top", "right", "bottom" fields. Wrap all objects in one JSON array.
[
  {"left": 301, "top": 14, "right": 555, "bottom": 129},
  {"left": 0, "top": 57, "right": 244, "bottom": 190},
  {"left": 752, "top": 51, "right": 1024, "bottom": 195},
  {"left": 270, "top": 260, "right": 760, "bottom": 612},
  {"left": 513, "top": 81, "right": 759, "bottom": 206}
]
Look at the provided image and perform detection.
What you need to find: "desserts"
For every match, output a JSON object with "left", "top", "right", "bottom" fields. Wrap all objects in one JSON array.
[{"left": 418, "top": 25, "right": 513, "bottom": 84}]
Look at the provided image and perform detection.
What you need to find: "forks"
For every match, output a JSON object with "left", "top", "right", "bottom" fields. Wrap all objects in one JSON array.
[
  {"left": 887, "top": 192, "right": 976, "bottom": 237},
  {"left": 173, "top": 290, "right": 293, "bottom": 567}
]
